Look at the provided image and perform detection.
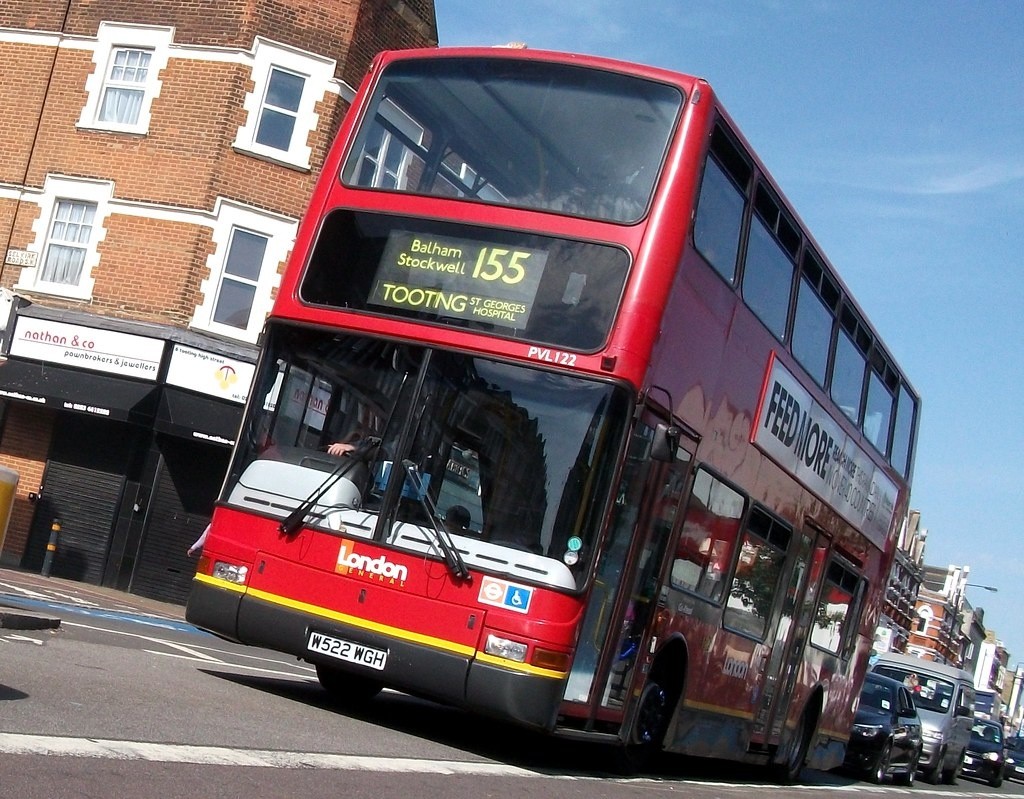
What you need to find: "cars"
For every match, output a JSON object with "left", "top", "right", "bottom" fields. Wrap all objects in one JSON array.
[
  {"left": 959, "top": 715, "right": 1012, "bottom": 787},
  {"left": 1001, "top": 736, "right": 1024, "bottom": 779},
  {"left": 842, "top": 670, "right": 925, "bottom": 787}
]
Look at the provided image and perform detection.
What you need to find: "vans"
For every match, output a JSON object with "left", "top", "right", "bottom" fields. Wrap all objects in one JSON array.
[{"left": 868, "top": 650, "right": 976, "bottom": 784}]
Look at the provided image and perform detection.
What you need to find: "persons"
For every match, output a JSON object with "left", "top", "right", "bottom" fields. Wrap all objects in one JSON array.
[
  {"left": 981, "top": 728, "right": 995, "bottom": 742},
  {"left": 187, "top": 523, "right": 211, "bottom": 556},
  {"left": 326, "top": 386, "right": 404, "bottom": 478}
]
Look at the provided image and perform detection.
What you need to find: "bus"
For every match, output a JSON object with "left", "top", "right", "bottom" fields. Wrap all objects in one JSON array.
[
  {"left": 184, "top": 40, "right": 926, "bottom": 776},
  {"left": 972, "top": 684, "right": 1016, "bottom": 739}
]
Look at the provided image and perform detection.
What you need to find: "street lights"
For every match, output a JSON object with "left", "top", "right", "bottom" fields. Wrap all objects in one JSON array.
[{"left": 942, "top": 583, "right": 999, "bottom": 668}]
